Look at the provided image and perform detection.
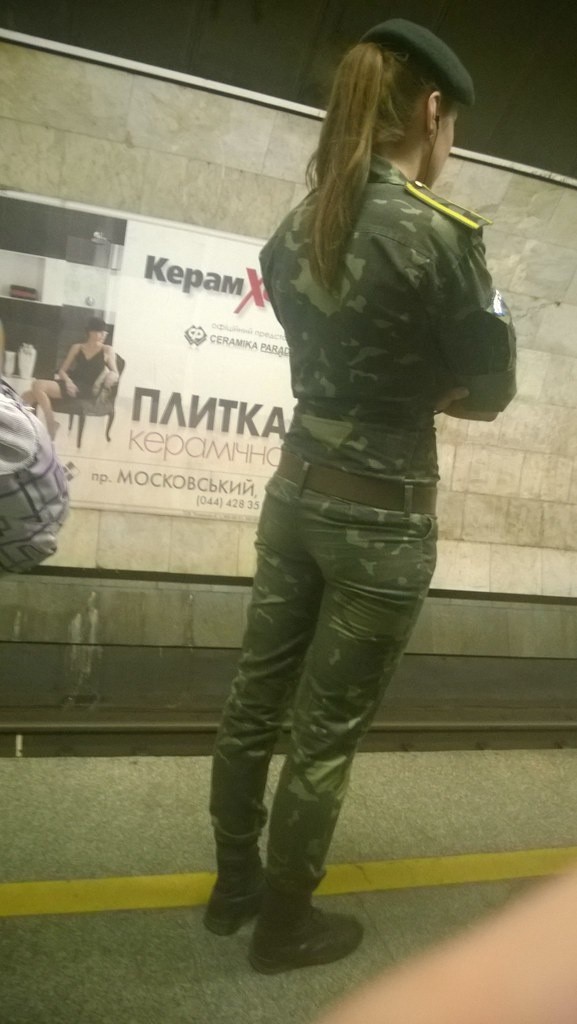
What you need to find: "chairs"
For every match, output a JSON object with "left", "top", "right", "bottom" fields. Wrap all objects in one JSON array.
[{"left": 33, "top": 353, "right": 124, "bottom": 447}]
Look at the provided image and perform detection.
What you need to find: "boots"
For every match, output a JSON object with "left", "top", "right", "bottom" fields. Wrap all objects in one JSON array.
[
  {"left": 205, "top": 817, "right": 270, "bottom": 934},
  {"left": 251, "top": 864, "right": 364, "bottom": 973}
]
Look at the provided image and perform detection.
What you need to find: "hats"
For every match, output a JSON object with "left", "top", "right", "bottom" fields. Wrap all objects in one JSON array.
[{"left": 360, "top": 17, "right": 476, "bottom": 104}]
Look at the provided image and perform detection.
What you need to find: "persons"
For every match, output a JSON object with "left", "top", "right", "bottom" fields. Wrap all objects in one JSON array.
[
  {"left": 20, "top": 317, "right": 120, "bottom": 441},
  {"left": 202, "top": 17, "right": 516, "bottom": 978}
]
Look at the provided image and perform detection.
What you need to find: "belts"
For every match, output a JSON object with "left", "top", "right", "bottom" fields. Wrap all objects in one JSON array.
[{"left": 276, "top": 452, "right": 438, "bottom": 516}]
